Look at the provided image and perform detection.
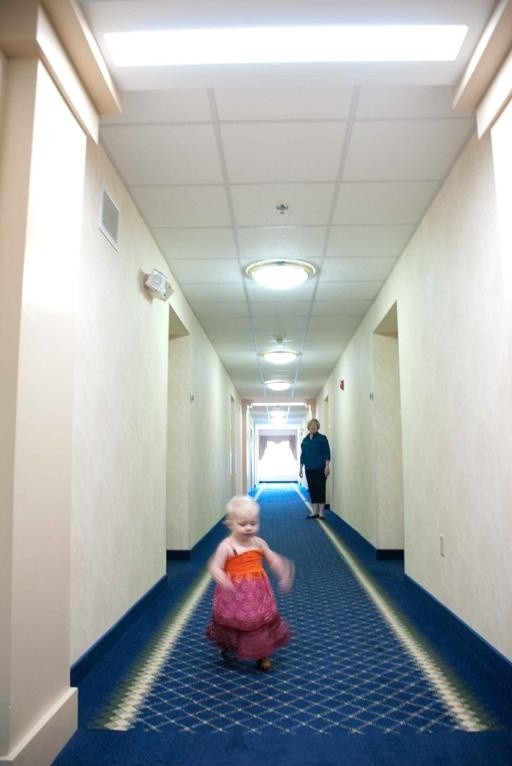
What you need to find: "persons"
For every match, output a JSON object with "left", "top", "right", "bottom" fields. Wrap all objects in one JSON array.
[
  {"left": 299, "top": 419, "right": 331, "bottom": 519},
  {"left": 204, "top": 495, "right": 296, "bottom": 670}
]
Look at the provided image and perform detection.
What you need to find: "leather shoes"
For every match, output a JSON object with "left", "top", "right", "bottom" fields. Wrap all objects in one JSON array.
[
  {"left": 318, "top": 513, "right": 325, "bottom": 520},
  {"left": 307, "top": 513, "right": 318, "bottom": 519}
]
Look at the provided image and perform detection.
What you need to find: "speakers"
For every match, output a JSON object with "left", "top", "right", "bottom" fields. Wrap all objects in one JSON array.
[{"left": 144, "top": 269, "right": 175, "bottom": 302}]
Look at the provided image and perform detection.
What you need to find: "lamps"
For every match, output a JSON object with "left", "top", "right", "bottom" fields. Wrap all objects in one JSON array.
[
  {"left": 262, "top": 377, "right": 294, "bottom": 391},
  {"left": 245, "top": 258, "right": 315, "bottom": 288},
  {"left": 256, "top": 349, "right": 301, "bottom": 364}
]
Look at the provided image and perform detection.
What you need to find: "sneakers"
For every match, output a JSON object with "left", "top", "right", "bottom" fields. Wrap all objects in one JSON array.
[
  {"left": 257, "top": 658, "right": 272, "bottom": 671},
  {"left": 221, "top": 650, "right": 235, "bottom": 662}
]
